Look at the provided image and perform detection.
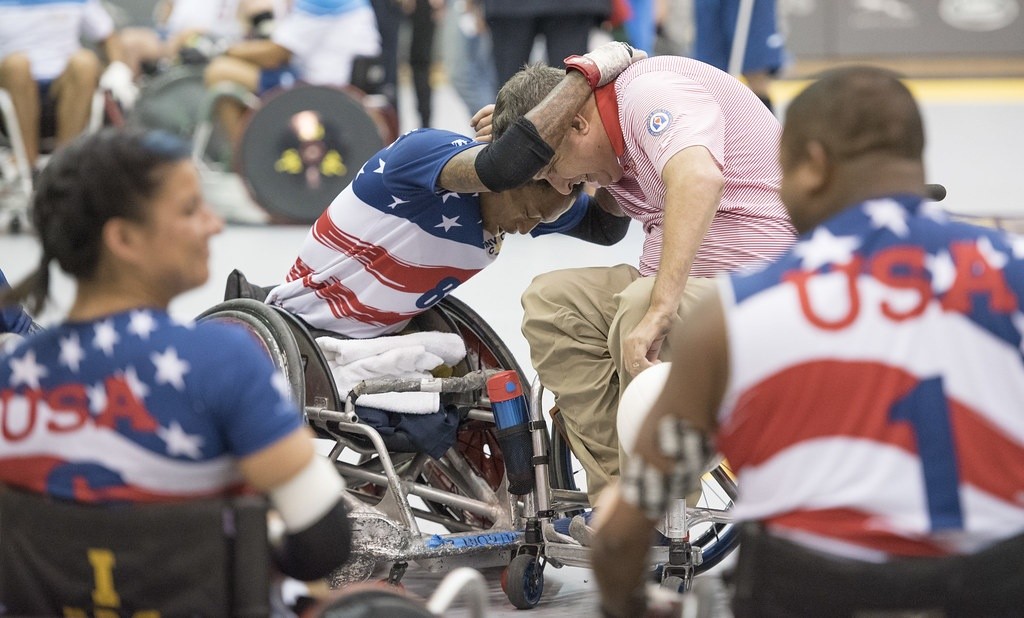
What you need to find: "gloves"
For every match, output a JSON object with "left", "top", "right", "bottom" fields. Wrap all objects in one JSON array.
[{"left": 564, "top": 41, "right": 648, "bottom": 91}]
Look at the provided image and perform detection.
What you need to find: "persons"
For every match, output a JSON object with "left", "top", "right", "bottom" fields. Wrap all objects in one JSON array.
[{"left": 0, "top": 0, "right": 1024, "bottom": 618}]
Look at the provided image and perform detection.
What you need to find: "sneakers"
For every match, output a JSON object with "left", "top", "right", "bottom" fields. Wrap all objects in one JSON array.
[
  {"left": 568, "top": 511, "right": 689, "bottom": 547},
  {"left": 545, "top": 516, "right": 579, "bottom": 545}
]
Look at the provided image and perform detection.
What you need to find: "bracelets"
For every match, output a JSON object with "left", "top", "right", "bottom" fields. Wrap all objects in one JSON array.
[{"left": 599, "top": 595, "right": 649, "bottom": 618}]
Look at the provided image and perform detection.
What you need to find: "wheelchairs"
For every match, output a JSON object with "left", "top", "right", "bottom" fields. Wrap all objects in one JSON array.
[
  {"left": 3, "top": 271, "right": 1024, "bottom": 616},
  {"left": 1, "top": 43, "right": 387, "bottom": 245}
]
